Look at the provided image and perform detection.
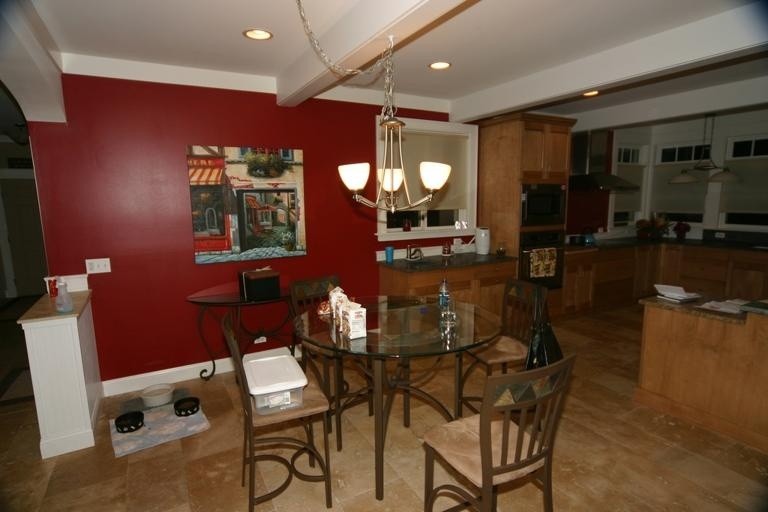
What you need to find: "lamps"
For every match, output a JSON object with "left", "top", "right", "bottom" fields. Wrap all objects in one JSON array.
[
  {"left": 665, "top": 111, "right": 745, "bottom": 185},
  {"left": 298, "top": 1, "right": 453, "bottom": 215}
]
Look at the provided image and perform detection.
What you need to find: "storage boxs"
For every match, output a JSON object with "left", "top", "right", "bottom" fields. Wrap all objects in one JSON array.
[{"left": 240, "top": 346, "right": 309, "bottom": 417}]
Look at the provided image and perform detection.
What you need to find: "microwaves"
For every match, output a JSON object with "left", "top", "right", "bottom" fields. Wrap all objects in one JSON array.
[{"left": 521, "top": 183, "right": 566, "bottom": 227}]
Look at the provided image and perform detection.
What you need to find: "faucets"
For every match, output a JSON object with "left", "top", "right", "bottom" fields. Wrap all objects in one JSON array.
[
  {"left": 406, "top": 243, "right": 426, "bottom": 260},
  {"left": 407, "top": 259, "right": 425, "bottom": 274}
]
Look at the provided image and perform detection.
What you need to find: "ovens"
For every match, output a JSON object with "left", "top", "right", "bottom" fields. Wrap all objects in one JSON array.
[{"left": 519, "top": 230, "right": 565, "bottom": 291}]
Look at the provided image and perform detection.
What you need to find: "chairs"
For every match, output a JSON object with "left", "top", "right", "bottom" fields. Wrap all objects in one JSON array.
[
  {"left": 285, "top": 272, "right": 373, "bottom": 436},
  {"left": 220, "top": 310, "right": 332, "bottom": 512},
  {"left": 422, "top": 352, "right": 578, "bottom": 511},
  {"left": 454, "top": 276, "right": 548, "bottom": 420}
]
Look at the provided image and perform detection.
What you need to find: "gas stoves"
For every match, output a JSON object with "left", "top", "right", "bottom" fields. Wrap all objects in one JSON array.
[{"left": 590, "top": 226, "right": 637, "bottom": 241}]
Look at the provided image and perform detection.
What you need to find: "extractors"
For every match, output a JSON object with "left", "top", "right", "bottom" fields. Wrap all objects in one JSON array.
[{"left": 569, "top": 128, "right": 640, "bottom": 193}]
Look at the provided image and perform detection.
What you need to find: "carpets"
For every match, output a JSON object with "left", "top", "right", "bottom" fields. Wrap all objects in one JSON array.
[{"left": 109, "top": 401, "right": 212, "bottom": 459}]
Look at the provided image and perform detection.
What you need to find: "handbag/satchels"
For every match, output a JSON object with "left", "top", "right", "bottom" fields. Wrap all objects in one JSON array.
[{"left": 526, "top": 286, "right": 563, "bottom": 373}]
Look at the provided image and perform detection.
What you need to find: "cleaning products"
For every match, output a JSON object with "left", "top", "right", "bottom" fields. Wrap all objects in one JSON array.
[{"left": 53, "top": 274, "right": 72, "bottom": 313}]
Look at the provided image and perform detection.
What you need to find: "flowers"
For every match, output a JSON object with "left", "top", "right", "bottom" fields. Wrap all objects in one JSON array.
[{"left": 636, "top": 212, "right": 674, "bottom": 236}]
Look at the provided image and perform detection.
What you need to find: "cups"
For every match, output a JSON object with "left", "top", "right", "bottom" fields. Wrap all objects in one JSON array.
[{"left": 385, "top": 247, "right": 394, "bottom": 263}]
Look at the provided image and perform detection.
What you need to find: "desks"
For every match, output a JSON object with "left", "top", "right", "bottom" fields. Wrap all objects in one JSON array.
[
  {"left": 378, "top": 251, "right": 517, "bottom": 337},
  {"left": 184, "top": 279, "right": 295, "bottom": 381}
]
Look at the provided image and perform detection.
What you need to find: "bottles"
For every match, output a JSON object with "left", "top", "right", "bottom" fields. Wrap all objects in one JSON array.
[{"left": 438, "top": 274, "right": 453, "bottom": 311}]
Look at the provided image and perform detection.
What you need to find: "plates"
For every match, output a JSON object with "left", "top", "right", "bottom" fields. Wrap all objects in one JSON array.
[{"left": 694, "top": 298, "right": 750, "bottom": 315}]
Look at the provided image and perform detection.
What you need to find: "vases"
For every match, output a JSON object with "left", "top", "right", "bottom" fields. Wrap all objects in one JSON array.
[{"left": 637, "top": 230, "right": 664, "bottom": 240}]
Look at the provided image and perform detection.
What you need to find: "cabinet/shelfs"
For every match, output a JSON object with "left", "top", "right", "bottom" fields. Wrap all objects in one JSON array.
[
  {"left": 639, "top": 292, "right": 767, "bottom": 451},
  {"left": 476, "top": 111, "right": 569, "bottom": 323},
  {"left": 564, "top": 241, "right": 766, "bottom": 313},
  {"left": 17, "top": 289, "right": 103, "bottom": 461}
]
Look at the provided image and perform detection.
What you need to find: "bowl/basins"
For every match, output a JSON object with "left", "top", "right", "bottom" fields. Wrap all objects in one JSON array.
[
  {"left": 114, "top": 411, "right": 145, "bottom": 432},
  {"left": 138, "top": 382, "right": 177, "bottom": 408},
  {"left": 172, "top": 396, "right": 202, "bottom": 417}
]
